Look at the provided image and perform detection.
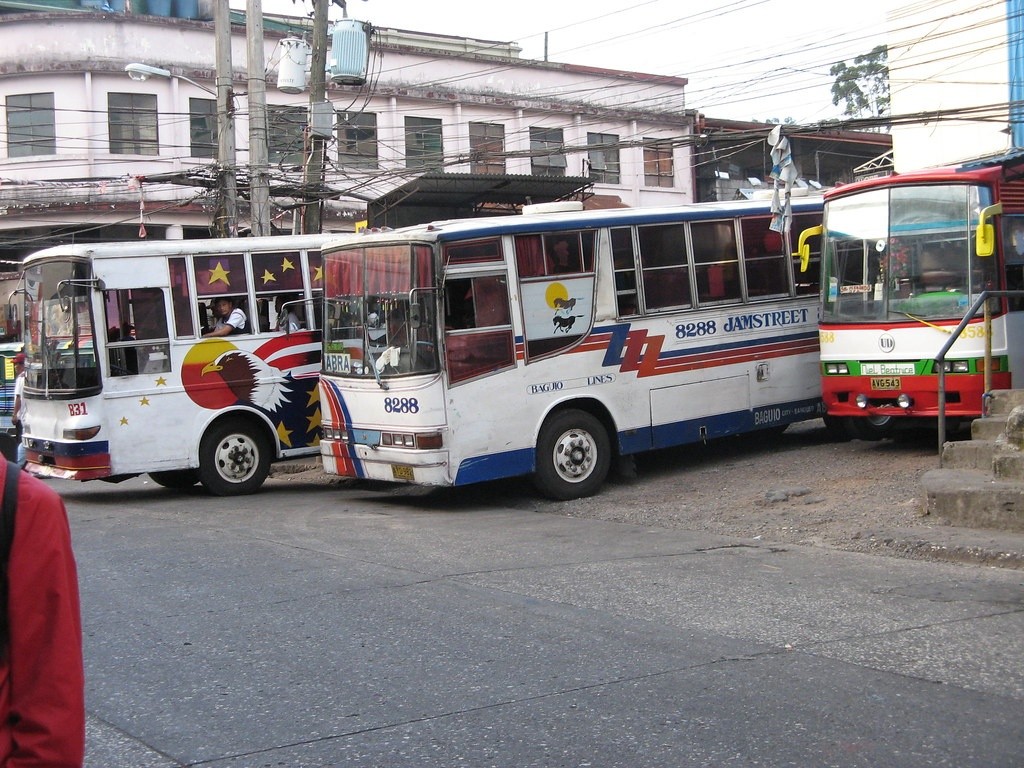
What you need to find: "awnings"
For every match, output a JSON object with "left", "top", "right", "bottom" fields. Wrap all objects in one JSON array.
[{"left": 368, "top": 172, "right": 596, "bottom": 209}]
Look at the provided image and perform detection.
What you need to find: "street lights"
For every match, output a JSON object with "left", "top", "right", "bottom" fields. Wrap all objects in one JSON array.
[{"left": 124, "top": 63, "right": 237, "bottom": 237}]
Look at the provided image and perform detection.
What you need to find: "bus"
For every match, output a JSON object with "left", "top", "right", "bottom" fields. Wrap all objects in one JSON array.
[
  {"left": 278, "top": 183, "right": 889, "bottom": 504},
  {"left": 7, "top": 231, "right": 365, "bottom": 498},
  {"left": 806, "top": 164, "right": 1023, "bottom": 442}
]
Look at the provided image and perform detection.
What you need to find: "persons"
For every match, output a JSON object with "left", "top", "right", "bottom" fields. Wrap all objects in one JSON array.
[
  {"left": 202, "top": 297, "right": 251, "bottom": 338},
  {"left": 0, "top": 452, "right": 86, "bottom": 768},
  {"left": 11, "top": 353, "right": 26, "bottom": 467},
  {"left": 275, "top": 294, "right": 301, "bottom": 331}
]
